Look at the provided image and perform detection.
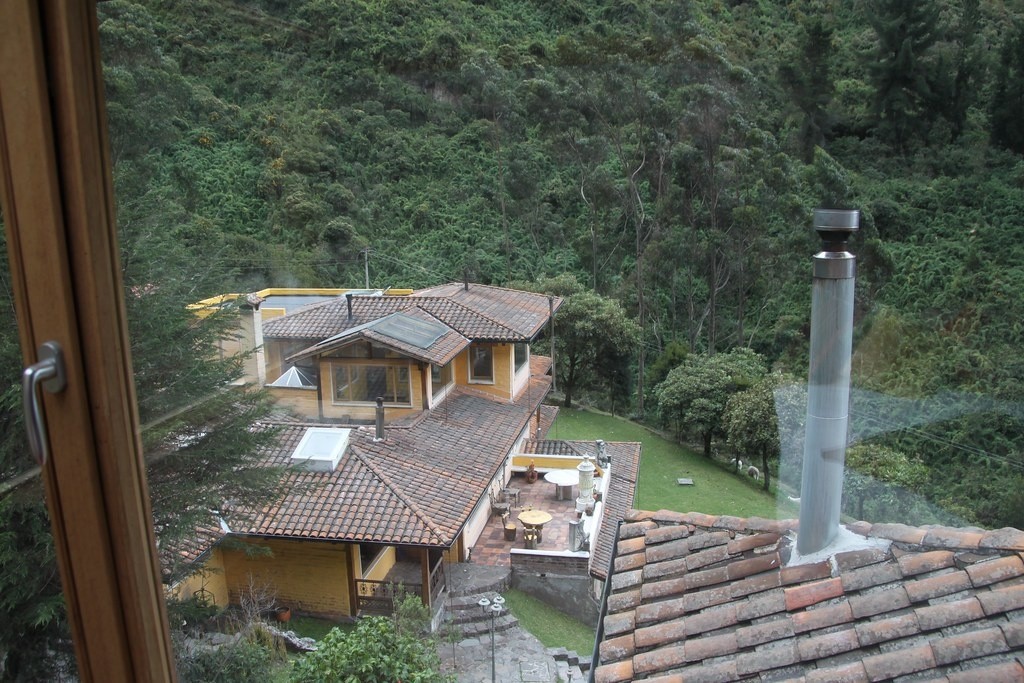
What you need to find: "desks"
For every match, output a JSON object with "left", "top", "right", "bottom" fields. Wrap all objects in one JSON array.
[
  {"left": 517, "top": 510, "right": 553, "bottom": 543},
  {"left": 544, "top": 470, "right": 580, "bottom": 500}
]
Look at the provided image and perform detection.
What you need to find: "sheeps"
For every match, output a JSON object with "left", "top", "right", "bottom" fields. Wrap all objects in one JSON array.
[
  {"left": 747, "top": 465, "right": 760, "bottom": 481},
  {"left": 788, "top": 496, "right": 801, "bottom": 503},
  {"left": 730, "top": 458, "right": 742, "bottom": 470}
]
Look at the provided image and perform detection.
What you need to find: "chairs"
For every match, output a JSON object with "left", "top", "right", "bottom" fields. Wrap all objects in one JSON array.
[
  {"left": 488, "top": 488, "right": 511, "bottom": 523},
  {"left": 497, "top": 475, "right": 521, "bottom": 508},
  {"left": 520, "top": 504, "right": 532, "bottom": 512},
  {"left": 501, "top": 510, "right": 517, "bottom": 541},
  {"left": 523, "top": 527, "right": 538, "bottom": 549}
]
